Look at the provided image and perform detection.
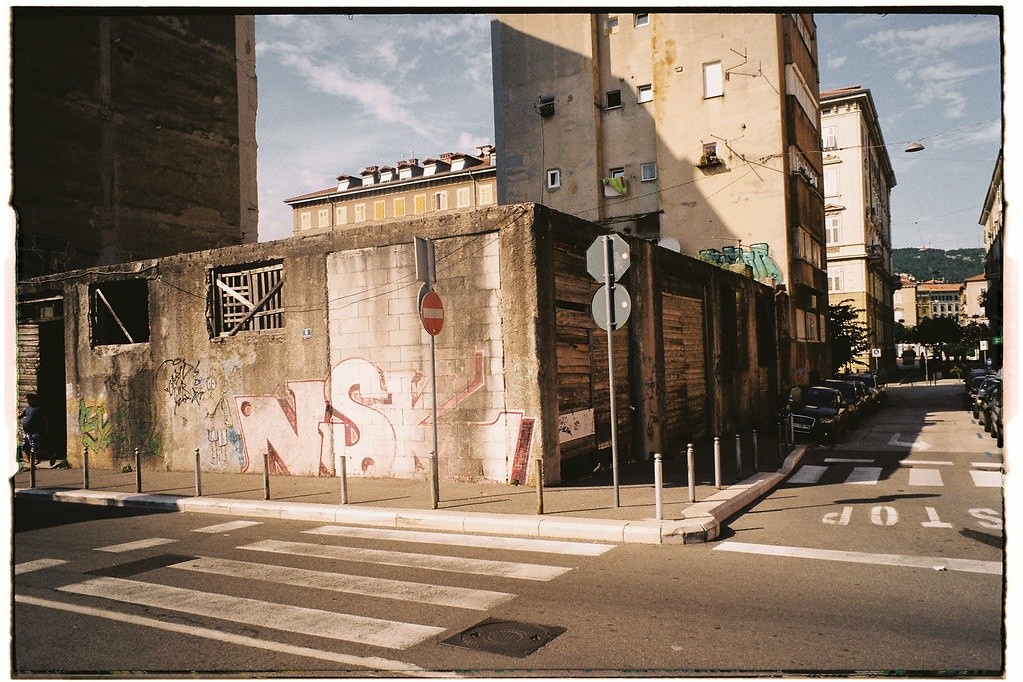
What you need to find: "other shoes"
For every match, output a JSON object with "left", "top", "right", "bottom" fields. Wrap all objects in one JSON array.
[
  {"left": 23, "top": 465, "right": 36, "bottom": 472},
  {"left": 50, "top": 449, "right": 57, "bottom": 466}
]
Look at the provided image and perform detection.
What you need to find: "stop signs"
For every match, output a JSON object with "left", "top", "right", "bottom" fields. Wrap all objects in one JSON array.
[{"left": 421, "top": 291, "right": 445, "bottom": 336}]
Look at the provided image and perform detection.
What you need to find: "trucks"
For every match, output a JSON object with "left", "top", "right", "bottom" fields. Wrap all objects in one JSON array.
[{"left": 903, "top": 351, "right": 916, "bottom": 365}]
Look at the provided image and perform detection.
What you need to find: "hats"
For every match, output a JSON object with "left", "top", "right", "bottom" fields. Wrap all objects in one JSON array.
[{"left": 25, "top": 394, "right": 35, "bottom": 398}]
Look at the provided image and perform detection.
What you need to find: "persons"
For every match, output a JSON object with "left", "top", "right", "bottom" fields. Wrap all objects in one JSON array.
[{"left": 919, "top": 352, "right": 925, "bottom": 377}]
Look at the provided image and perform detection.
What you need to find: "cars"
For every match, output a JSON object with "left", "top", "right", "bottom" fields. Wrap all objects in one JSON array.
[
  {"left": 967, "top": 368, "right": 997, "bottom": 403},
  {"left": 973, "top": 378, "right": 1003, "bottom": 449},
  {"left": 823, "top": 372, "right": 888, "bottom": 429},
  {"left": 787, "top": 387, "right": 844, "bottom": 443}
]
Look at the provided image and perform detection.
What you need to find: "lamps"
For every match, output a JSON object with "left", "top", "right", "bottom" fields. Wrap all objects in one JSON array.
[{"left": 905, "top": 140, "right": 926, "bottom": 153}]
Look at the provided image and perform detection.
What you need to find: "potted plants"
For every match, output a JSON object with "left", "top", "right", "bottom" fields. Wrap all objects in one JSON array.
[{"left": 540, "top": 103, "right": 554, "bottom": 117}]
